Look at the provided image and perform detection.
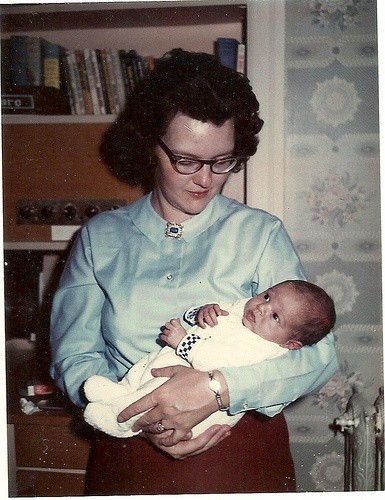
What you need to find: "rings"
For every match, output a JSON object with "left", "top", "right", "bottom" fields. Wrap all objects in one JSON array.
[{"left": 157, "top": 420, "right": 167, "bottom": 433}]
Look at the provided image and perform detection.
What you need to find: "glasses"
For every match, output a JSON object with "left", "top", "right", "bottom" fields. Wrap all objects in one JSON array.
[{"left": 158, "top": 139, "right": 239, "bottom": 174}]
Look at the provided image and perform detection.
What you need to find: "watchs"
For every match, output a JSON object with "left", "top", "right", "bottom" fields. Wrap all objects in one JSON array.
[{"left": 208, "top": 371, "right": 224, "bottom": 409}]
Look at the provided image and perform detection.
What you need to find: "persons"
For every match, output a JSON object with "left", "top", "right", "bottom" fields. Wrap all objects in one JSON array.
[
  {"left": 84, "top": 280, "right": 337, "bottom": 441},
  {"left": 50, "top": 48, "right": 341, "bottom": 498}
]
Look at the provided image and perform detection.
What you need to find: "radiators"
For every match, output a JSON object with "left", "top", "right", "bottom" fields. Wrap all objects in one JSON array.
[{"left": 332, "top": 385, "right": 385, "bottom": 491}]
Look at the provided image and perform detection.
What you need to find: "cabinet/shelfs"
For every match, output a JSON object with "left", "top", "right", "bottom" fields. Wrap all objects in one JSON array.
[{"left": 0, "top": 4, "right": 254, "bottom": 498}]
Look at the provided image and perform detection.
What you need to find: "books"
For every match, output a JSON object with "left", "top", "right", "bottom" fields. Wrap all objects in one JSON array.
[
  {"left": 64, "top": 49, "right": 154, "bottom": 115},
  {"left": 217, "top": 38, "right": 246, "bottom": 76},
  {"left": 0, "top": 36, "right": 64, "bottom": 87}
]
